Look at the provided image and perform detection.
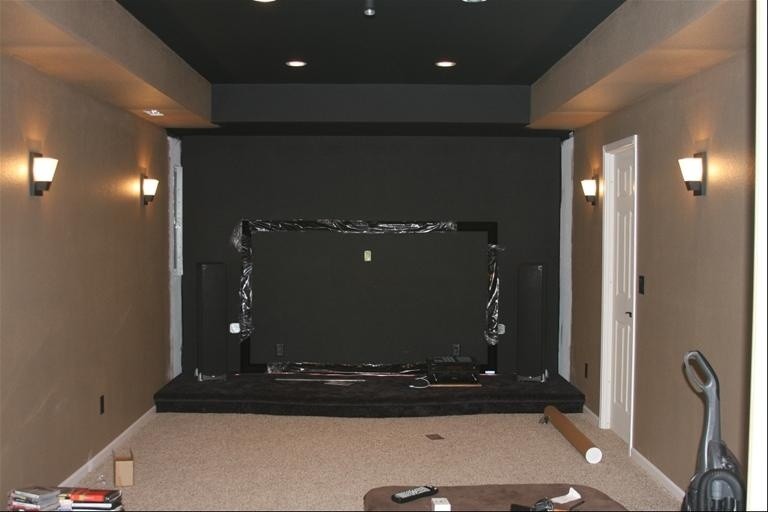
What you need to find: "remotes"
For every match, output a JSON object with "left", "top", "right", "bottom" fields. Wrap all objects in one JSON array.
[{"left": 392, "top": 483, "right": 438, "bottom": 504}]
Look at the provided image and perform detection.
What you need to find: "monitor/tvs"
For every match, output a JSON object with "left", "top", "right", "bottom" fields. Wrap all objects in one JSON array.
[{"left": 236, "top": 217, "right": 499, "bottom": 385}]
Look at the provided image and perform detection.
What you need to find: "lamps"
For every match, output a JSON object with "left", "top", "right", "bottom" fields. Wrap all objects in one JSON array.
[
  {"left": 678, "top": 151, "right": 707, "bottom": 196},
  {"left": 28, "top": 150, "right": 58, "bottom": 196},
  {"left": 580, "top": 175, "right": 598, "bottom": 206},
  {"left": 140, "top": 173, "right": 160, "bottom": 209}
]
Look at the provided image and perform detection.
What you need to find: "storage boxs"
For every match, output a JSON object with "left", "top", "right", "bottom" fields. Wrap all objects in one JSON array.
[{"left": 111, "top": 449, "right": 134, "bottom": 488}]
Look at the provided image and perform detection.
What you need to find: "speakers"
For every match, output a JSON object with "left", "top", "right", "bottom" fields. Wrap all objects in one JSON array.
[
  {"left": 193, "top": 261, "right": 228, "bottom": 383},
  {"left": 516, "top": 263, "right": 550, "bottom": 384}
]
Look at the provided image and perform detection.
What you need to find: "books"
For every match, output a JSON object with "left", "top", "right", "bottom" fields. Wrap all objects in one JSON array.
[{"left": 8, "top": 486, "right": 124, "bottom": 511}]
